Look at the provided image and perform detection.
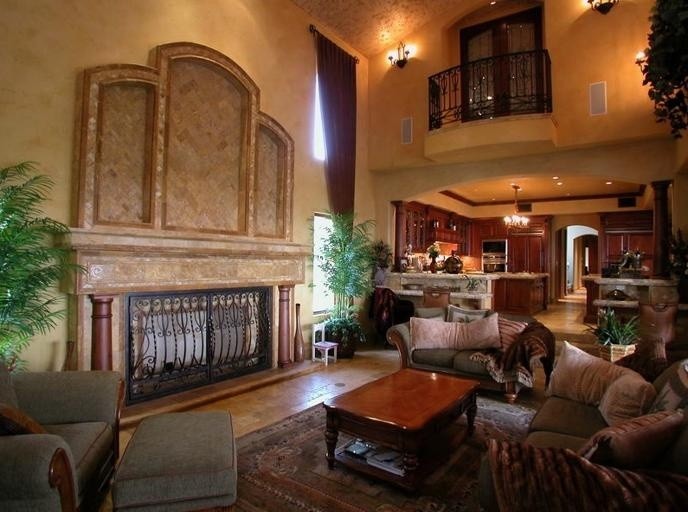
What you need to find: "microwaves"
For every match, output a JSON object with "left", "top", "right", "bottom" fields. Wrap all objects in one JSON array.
[{"left": 482, "top": 238, "right": 508, "bottom": 256}]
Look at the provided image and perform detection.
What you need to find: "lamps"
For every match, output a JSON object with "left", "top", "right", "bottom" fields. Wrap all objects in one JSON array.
[
  {"left": 635, "top": 52, "right": 646, "bottom": 73},
  {"left": 388, "top": 41, "right": 409, "bottom": 68},
  {"left": 587, "top": 0, "right": 619, "bottom": 15},
  {"left": 503, "top": 185, "right": 528, "bottom": 227}
]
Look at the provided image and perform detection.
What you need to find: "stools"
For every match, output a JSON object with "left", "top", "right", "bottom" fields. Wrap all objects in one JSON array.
[{"left": 111, "top": 409, "right": 238, "bottom": 512}]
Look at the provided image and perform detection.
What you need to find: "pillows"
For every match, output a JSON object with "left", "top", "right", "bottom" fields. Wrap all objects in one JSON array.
[
  {"left": 0, "top": 404, "right": 49, "bottom": 436},
  {"left": 577, "top": 409, "right": 686, "bottom": 470},
  {"left": 497, "top": 318, "right": 529, "bottom": 353},
  {"left": 646, "top": 362, "right": 688, "bottom": 413},
  {"left": 447, "top": 304, "right": 488, "bottom": 324},
  {"left": 545, "top": 341, "right": 657, "bottom": 427},
  {"left": 410, "top": 312, "right": 502, "bottom": 350}
]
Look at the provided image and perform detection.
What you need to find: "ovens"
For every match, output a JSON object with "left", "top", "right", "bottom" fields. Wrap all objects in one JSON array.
[{"left": 482, "top": 256, "right": 507, "bottom": 273}]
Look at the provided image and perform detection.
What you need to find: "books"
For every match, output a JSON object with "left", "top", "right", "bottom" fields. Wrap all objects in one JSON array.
[{"left": 365, "top": 451, "right": 406, "bottom": 476}]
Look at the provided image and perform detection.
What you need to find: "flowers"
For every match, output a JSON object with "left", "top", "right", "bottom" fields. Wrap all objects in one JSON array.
[{"left": 427, "top": 243, "right": 441, "bottom": 258}]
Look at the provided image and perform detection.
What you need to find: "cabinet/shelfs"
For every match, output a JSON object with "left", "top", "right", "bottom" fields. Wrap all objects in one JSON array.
[
  {"left": 390, "top": 199, "right": 471, "bottom": 273},
  {"left": 598, "top": 211, "right": 654, "bottom": 275},
  {"left": 471, "top": 215, "right": 552, "bottom": 274}
]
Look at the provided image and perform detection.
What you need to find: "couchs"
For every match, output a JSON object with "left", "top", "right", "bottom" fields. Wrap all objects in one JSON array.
[
  {"left": 386, "top": 309, "right": 555, "bottom": 393},
  {"left": 477, "top": 343, "right": 688, "bottom": 512}
]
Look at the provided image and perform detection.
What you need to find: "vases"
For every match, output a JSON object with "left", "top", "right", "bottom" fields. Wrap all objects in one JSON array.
[{"left": 429, "top": 258, "right": 437, "bottom": 274}]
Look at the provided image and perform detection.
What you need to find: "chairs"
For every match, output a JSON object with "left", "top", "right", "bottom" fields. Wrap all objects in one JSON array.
[
  {"left": 312, "top": 323, "right": 339, "bottom": 367},
  {"left": 1, "top": 362, "right": 126, "bottom": 512}
]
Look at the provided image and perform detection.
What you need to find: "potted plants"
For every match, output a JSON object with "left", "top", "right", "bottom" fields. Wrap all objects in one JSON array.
[
  {"left": 465, "top": 274, "right": 482, "bottom": 294},
  {"left": 306, "top": 208, "right": 379, "bottom": 359},
  {"left": 588, "top": 305, "right": 641, "bottom": 363}
]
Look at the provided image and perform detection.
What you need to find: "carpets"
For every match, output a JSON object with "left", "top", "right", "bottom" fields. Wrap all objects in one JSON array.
[{"left": 235, "top": 394, "right": 543, "bottom": 512}]
